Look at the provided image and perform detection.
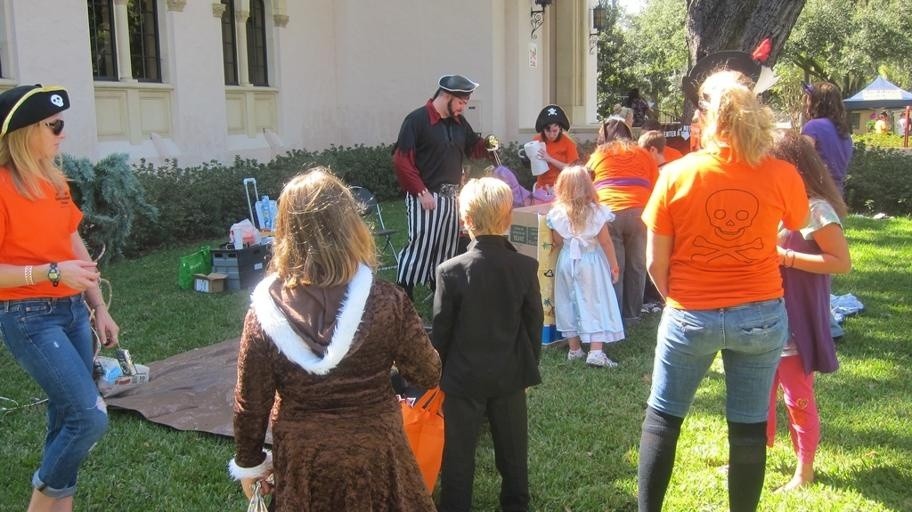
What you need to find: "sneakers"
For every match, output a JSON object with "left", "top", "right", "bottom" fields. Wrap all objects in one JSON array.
[
  {"left": 565, "top": 350, "right": 589, "bottom": 362},
  {"left": 641, "top": 302, "right": 661, "bottom": 313},
  {"left": 586, "top": 352, "right": 621, "bottom": 370}
]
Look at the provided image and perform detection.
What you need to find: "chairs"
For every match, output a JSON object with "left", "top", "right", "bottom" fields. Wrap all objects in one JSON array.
[{"left": 347, "top": 187, "right": 400, "bottom": 272}]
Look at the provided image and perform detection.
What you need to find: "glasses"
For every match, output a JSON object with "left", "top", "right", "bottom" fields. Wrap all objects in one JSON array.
[{"left": 35, "top": 119, "right": 66, "bottom": 135}]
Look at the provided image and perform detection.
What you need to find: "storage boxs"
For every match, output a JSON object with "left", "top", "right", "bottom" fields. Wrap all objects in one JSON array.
[
  {"left": 192, "top": 272, "right": 227, "bottom": 294},
  {"left": 211, "top": 242, "right": 266, "bottom": 267},
  {"left": 509, "top": 203, "right": 564, "bottom": 346},
  {"left": 212, "top": 266, "right": 266, "bottom": 290}
]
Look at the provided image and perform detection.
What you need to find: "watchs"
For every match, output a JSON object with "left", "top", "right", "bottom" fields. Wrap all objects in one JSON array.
[{"left": 47, "top": 262, "right": 60, "bottom": 287}]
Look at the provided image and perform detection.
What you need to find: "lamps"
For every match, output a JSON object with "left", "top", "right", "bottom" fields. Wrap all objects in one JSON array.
[
  {"left": 590, "top": 1, "right": 607, "bottom": 54},
  {"left": 531, "top": 0, "right": 553, "bottom": 38}
]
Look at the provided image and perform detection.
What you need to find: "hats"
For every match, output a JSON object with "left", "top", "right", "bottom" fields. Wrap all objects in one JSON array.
[
  {"left": 534, "top": 104, "right": 570, "bottom": 135},
  {"left": 0, "top": 81, "right": 70, "bottom": 137},
  {"left": 436, "top": 74, "right": 479, "bottom": 97}
]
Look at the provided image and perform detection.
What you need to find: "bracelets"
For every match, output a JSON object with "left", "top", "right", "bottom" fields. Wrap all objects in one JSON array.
[
  {"left": 25, "top": 265, "right": 36, "bottom": 285},
  {"left": 94, "top": 303, "right": 105, "bottom": 307}
]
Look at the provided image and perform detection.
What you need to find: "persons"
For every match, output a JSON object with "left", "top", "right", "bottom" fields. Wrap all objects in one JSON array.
[
  {"left": 638, "top": 71, "right": 809, "bottom": 512},
  {"left": 772, "top": 129, "right": 850, "bottom": 495},
  {"left": 586, "top": 67, "right": 864, "bottom": 336},
  {"left": 0, "top": 84, "right": 119, "bottom": 512},
  {"left": 431, "top": 176, "right": 545, "bottom": 512},
  {"left": 865, "top": 110, "right": 912, "bottom": 139},
  {"left": 545, "top": 165, "right": 626, "bottom": 368},
  {"left": 228, "top": 163, "right": 442, "bottom": 512},
  {"left": 391, "top": 74, "right": 503, "bottom": 302},
  {"left": 532, "top": 104, "right": 579, "bottom": 192}
]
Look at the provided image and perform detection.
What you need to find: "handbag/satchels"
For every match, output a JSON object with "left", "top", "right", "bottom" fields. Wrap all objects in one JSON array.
[
  {"left": 230, "top": 219, "right": 260, "bottom": 250},
  {"left": 395, "top": 386, "right": 446, "bottom": 497},
  {"left": 176, "top": 245, "right": 214, "bottom": 291}
]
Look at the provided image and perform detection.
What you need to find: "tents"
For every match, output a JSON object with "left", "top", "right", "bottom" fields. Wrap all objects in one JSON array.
[{"left": 842, "top": 76, "right": 911, "bottom": 112}]
